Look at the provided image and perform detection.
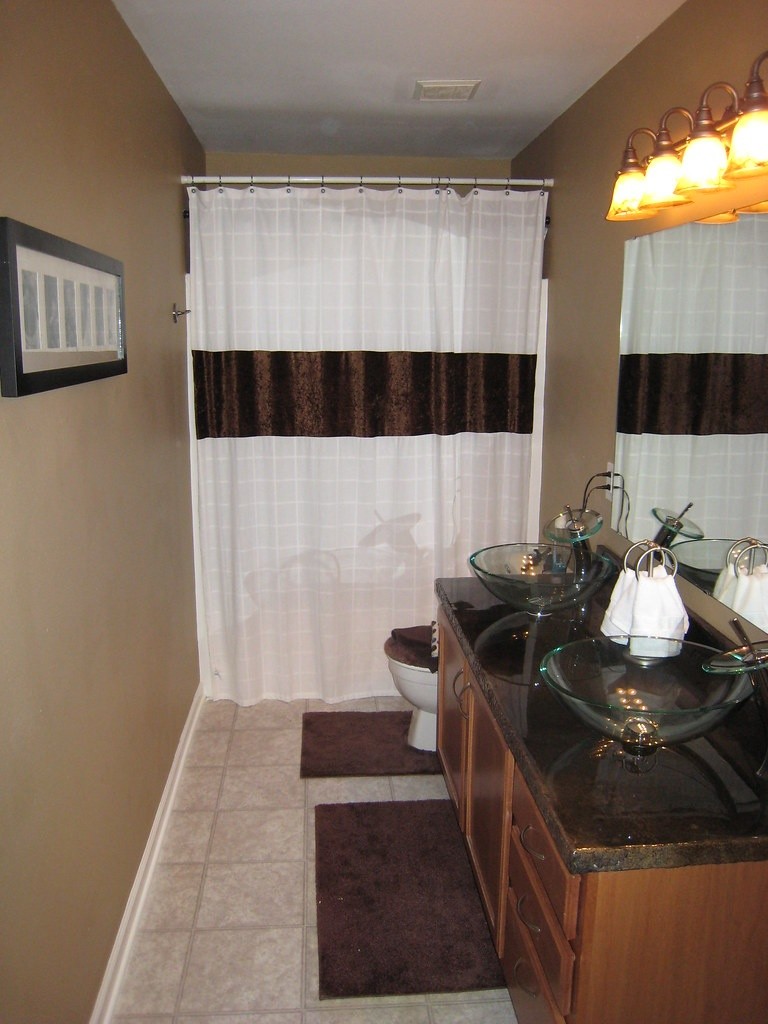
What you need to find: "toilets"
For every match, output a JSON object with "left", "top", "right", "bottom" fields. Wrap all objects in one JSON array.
[{"left": 383, "top": 623, "right": 439, "bottom": 753}]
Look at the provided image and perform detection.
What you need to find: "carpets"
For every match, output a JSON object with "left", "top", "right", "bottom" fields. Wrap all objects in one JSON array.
[
  {"left": 314, "top": 798, "right": 507, "bottom": 1001},
  {"left": 300, "top": 711, "right": 441, "bottom": 773}
]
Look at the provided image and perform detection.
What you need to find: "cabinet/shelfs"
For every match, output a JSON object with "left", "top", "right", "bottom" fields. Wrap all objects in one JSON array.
[{"left": 437, "top": 601, "right": 768, "bottom": 1024}]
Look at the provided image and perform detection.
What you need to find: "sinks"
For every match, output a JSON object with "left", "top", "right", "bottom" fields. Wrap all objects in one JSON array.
[
  {"left": 537, "top": 636, "right": 761, "bottom": 751},
  {"left": 668, "top": 536, "right": 768, "bottom": 583},
  {"left": 468, "top": 540, "right": 615, "bottom": 616}
]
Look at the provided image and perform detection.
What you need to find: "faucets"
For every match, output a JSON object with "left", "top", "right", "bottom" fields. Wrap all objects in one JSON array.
[
  {"left": 728, "top": 615, "right": 768, "bottom": 664},
  {"left": 666, "top": 501, "right": 696, "bottom": 529},
  {"left": 564, "top": 503, "right": 584, "bottom": 530}
]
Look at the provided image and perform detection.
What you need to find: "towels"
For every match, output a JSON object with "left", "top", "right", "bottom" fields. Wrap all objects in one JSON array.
[
  {"left": 599, "top": 566, "right": 669, "bottom": 648},
  {"left": 608, "top": 574, "right": 691, "bottom": 658},
  {"left": 712, "top": 560, "right": 768, "bottom": 633}
]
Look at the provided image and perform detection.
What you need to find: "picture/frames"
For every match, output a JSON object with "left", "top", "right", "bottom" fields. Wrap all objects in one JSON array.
[{"left": 0, "top": 215, "right": 127, "bottom": 397}]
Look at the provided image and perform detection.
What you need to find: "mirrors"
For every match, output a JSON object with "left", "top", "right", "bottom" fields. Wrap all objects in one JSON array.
[{"left": 610, "top": 199, "right": 768, "bottom": 634}]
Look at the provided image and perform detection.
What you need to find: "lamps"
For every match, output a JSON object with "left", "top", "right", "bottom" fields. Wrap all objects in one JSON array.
[{"left": 605, "top": 51, "right": 768, "bottom": 225}]
